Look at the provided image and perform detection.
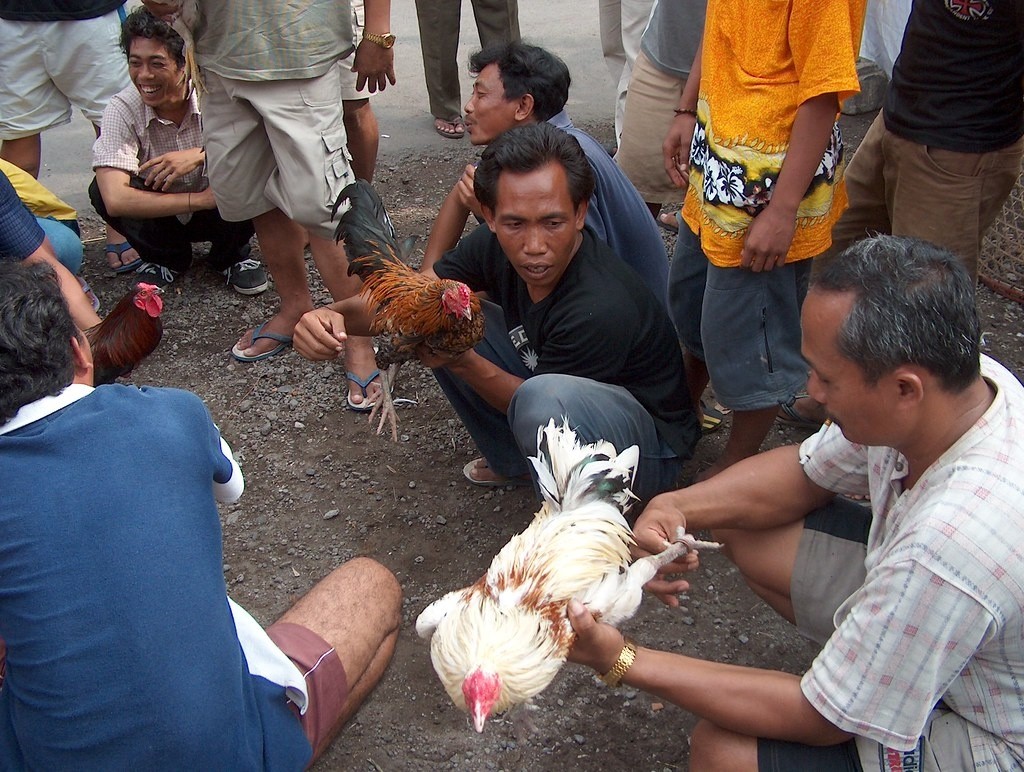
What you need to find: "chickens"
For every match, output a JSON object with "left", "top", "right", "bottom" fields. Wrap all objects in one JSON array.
[
  {"left": 414, "top": 416, "right": 724, "bottom": 735},
  {"left": 82, "top": 282, "right": 165, "bottom": 384},
  {"left": 330, "top": 179, "right": 485, "bottom": 440}
]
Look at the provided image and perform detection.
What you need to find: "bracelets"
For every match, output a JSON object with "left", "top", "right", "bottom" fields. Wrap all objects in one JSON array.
[
  {"left": 189, "top": 192, "right": 191, "bottom": 213},
  {"left": 673, "top": 107, "right": 697, "bottom": 117},
  {"left": 595, "top": 635, "right": 637, "bottom": 690},
  {"left": 317, "top": 305, "right": 332, "bottom": 311}
]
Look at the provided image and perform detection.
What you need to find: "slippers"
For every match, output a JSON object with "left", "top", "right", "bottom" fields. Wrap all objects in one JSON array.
[
  {"left": 230, "top": 317, "right": 292, "bottom": 361},
  {"left": 434, "top": 116, "right": 465, "bottom": 138},
  {"left": 462, "top": 455, "right": 542, "bottom": 489},
  {"left": 104, "top": 240, "right": 143, "bottom": 273},
  {"left": 774, "top": 392, "right": 821, "bottom": 431},
  {"left": 696, "top": 397, "right": 724, "bottom": 435},
  {"left": 656, "top": 208, "right": 682, "bottom": 235},
  {"left": 59, "top": 273, "right": 101, "bottom": 316},
  {"left": 345, "top": 370, "right": 390, "bottom": 412}
]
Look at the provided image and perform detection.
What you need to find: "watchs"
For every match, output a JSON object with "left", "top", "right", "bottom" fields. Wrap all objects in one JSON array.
[{"left": 361, "top": 28, "right": 396, "bottom": 49}]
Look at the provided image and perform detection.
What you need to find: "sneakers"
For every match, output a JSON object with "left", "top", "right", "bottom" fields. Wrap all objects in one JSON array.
[
  {"left": 129, "top": 262, "right": 181, "bottom": 294},
  {"left": 227, "top": 258, "right": 268, "bottom": 294}
]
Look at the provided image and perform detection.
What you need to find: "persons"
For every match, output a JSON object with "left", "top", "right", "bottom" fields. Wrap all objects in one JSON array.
[
  {"left": 774, "top": 0, "right": 1022, "bottom": 513},
  {"left": 660, "top": 0, "right": 869, "bottom": 485},
  {"left": 289, "top": 120, "right": 695, "bottom": 526},
  {"left": 86, "top": 3, "right": 269, "bottom": 295},
  {"left": 417, "top": 42, "right": 672, "bottom": 317},
  {"left": 304, "top": 0, "right": 382, "bottom": 251},
  {"left": 141, "top": 1, "right": 396, "bottom": 412},
  {"left": 414, "top": 0, "right": 525, "bottom": 139},
  {"left": 0, "top": 157, "right": 101, "bottom": 313},
  {"left": 0, "top": 167, "right": 102, "bottom": 331},
  {"left": 0, "top": 1, "right": 146, "bottom": 272},
  {"left": 606, "top": 0, "right": 707, "bottom": 233},
  {"left": 598, "top": 0, "right": 659, "bottom": 156},
  {"left": 558, "top": 234, "right": 1024, "bottom": 772},
  {"left": 0, "top": 259, "right": 409, "bottom": 771}
]
